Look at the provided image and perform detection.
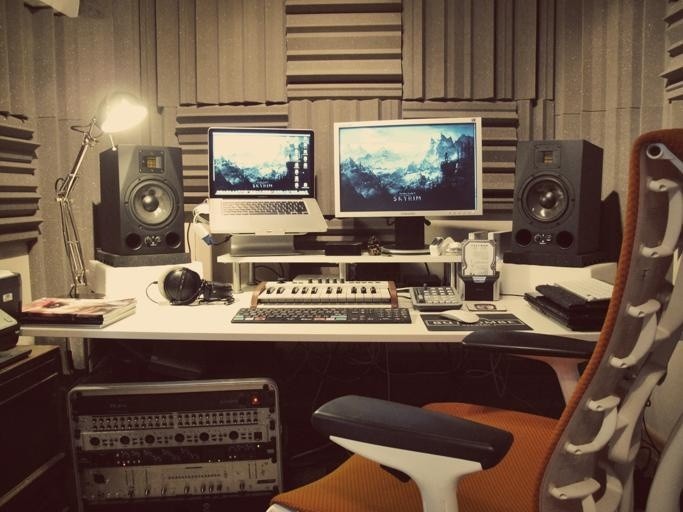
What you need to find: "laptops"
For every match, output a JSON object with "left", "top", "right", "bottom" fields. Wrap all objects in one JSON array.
[{"left": 207, "top": 126, "right": 328, "bottom": 234}]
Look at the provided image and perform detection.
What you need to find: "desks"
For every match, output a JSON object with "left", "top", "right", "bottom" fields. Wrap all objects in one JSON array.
[{"left": 20, "top": 245, "right": 623, "bottom": 407}]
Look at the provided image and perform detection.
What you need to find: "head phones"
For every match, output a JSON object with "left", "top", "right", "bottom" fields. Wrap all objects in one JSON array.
[{"left": 160, "top": 267, "right": 232, "bottom": 304}]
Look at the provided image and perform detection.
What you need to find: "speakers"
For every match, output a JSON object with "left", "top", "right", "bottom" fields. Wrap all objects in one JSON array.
[
  {"left": 511, "top": 139, "right": 603, "bottom": 254},
  {"left": 101, "top": 143, "right": 184, "bottom": 254}
]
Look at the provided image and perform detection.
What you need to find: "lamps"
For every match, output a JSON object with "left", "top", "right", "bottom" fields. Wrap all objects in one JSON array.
[{"left": 55, "top": 91, "right": 148, "bottom": 297}]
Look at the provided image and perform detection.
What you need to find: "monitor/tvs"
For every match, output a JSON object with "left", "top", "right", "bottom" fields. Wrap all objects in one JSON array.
[{"left": 333, "top": 116, "right": 483, "bottom": 253}]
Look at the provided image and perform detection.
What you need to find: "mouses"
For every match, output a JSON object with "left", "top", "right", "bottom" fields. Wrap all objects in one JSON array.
[{"left": 440, "top": 310, "right": 480, "bottom": 325}]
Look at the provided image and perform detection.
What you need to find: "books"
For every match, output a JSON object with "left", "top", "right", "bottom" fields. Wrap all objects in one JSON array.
[{"left": 21, "top": 297, "right": 137, "bottom": 329}]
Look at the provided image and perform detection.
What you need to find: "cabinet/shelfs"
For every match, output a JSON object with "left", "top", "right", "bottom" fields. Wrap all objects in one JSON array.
[{"left": 0, "top": 345, "right": 68, "bottom": 511}]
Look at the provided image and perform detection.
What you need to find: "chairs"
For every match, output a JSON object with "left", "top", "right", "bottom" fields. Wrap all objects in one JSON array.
[{"left": 264, "top": 128, "right": 682, "bottom": 511}]
